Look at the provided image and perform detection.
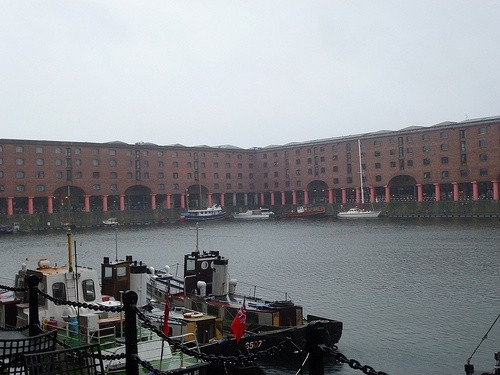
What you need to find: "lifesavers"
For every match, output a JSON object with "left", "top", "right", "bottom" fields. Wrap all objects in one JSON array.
[{"left": 183, "top": 312, "right": 204, "bottom": 318}]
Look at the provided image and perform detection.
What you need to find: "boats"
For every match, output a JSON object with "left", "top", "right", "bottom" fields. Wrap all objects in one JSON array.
[
  {"left": 284, "top": 205, "right": 326, "bottom": 218},
  {"left": 180, "top": 203, "right": 227, "bottom": 222},
  {"left": 231, "top": 207, "right": 275, "bottom": 221},
  {"left": 102, "top": 217, "right": 121, "bottom": 227}
]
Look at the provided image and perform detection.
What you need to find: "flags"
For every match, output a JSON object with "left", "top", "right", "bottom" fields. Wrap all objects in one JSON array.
[
  {"left": 230, "top": 305, "right": 246, "bottom": 344},
  {"left": 163, "top": 285, "right": 169, "bottom": 340}
]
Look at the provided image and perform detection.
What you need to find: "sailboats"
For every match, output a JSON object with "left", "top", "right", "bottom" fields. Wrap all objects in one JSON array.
[{"left": 337, "top": 139, "right": 382, "bottom": 219}]
